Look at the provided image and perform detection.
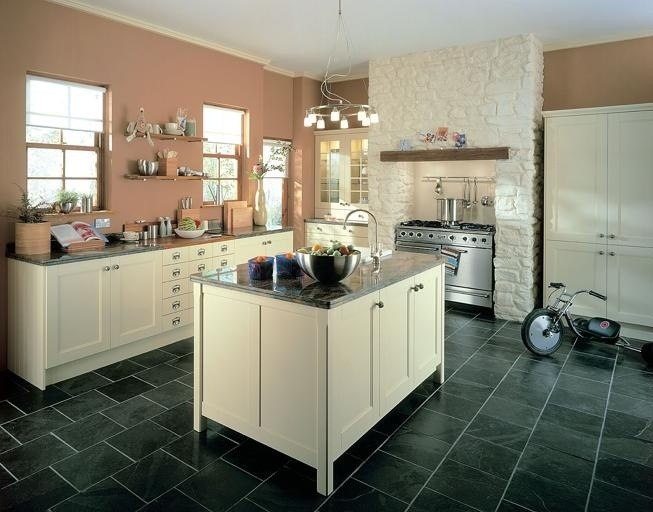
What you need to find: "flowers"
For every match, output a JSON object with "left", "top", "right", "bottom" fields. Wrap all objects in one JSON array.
[{"left": 248, "top": 138, "right": 298, "bottom": 178}]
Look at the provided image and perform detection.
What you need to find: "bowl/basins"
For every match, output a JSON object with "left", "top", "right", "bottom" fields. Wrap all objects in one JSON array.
[
  {"left": 298, "top": 282, "right": 353, "bottom": 303},
  {"left": 136, "top": 159, "right": 159, "bottom": 176},
  {"left": 295, "top": 246, "right": 362, "bottom": 284},
  {"left": 57, "top": 201, "right": 77, "bottom": 213},
  {"left": 173, "top": 229, "right": 205, "bottom": 239}
]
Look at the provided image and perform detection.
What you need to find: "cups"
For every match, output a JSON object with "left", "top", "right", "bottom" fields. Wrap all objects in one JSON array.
[
  {"left": 123, "top": 231, "right": 137, "bottom": 239},
  {"left": 275, "top": 280, "right": 302, "bottom": 294},
  {"left": 126, "top": 122, "right": 162, "bottom": 135},
  {"left": 274, "top": 255, "right": 300, "bottom": 279},
  {"left": 80, "top": 198, "right": 93, "bottom": 213},
  {"left": 248, "top": 280, "right": 273, "bottom": 290},
  {"left": 165, "top": 122, "right": 178, "bottom": 130},
  {"left": 249, "top": 255, "right": 273, "bottom": 281}
]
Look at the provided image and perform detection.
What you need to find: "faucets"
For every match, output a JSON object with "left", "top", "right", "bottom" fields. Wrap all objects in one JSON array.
[{"left": 343, "top": 209, "right": 383, "bottom": 272}]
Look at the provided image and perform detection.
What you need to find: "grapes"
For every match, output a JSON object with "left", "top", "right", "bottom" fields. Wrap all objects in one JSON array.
[{"left": 304, "top": 242, "right": 353, "bottom": 256}]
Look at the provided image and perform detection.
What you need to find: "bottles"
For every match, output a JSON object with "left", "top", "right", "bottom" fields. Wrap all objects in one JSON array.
[
  {"left": 181, "top": 196, "right": 192, "bottom": 209},
  {"left": 135, "top": 217, "right": 172, "bottom": 240},
  {"left": 176, "top": 104, "right": 196, "bottom": 136},
  {"left": 136, "top": 107, "right": 146, "bottom": 135}
]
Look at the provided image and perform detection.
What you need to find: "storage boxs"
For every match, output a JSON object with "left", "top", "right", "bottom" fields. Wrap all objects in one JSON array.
[
  {"left": 175, "top": 207, "right": 200, "bottom": 219},
  {"left": 156, "top": 157, "right": 178, "bottom": 177}
]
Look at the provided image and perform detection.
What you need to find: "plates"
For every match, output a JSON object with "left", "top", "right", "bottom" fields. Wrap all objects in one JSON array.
[
  {"left": 121, "top": 238, "right": 138, "bottom": 242},
  {"left": 163, "top": 130, "right": 183, "bottom": 135},
  {"left": 324, "top": 215, "right": 336, "bottom": 222}
]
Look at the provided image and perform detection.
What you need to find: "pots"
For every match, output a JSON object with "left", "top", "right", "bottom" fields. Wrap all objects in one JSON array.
[{"left": 435, "top": 198, "right": 464, "bottom": 222}]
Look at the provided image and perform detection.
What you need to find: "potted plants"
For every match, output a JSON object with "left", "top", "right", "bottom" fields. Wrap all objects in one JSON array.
[
  {"left": 2, "top": 185, "right": 57, "bottom": 255},
  {"left": 56, "top": 191, "right": 79, "bottom": 213}
]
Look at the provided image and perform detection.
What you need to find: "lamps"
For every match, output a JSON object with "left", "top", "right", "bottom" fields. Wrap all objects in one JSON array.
[{"left": 304, "top": 1, "right": 378, "bottom": 130}]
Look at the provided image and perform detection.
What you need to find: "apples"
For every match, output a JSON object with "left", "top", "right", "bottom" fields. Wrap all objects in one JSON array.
[{"left": 256, "top": 256, "right": 266, "bottom": 263}]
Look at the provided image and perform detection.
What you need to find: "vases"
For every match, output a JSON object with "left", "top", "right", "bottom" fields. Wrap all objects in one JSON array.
[{"left": 252, "top": 178, "right": 268, "bottom": 227}]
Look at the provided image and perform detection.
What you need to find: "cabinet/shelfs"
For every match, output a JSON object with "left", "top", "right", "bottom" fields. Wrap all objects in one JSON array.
[
  {"left": 311, "top": 126, "right": 368, "bottom": 222},
  {"left": 119, "top": 130, "right": 211, "bottom": 184},
  {"left": 538, "top": 103, "right": 653, "bottom": 249},
  {"left": 161, "top": 239, "right": 236, "bottom": 348},
  {"left": 334, "top": 222, "right": 369, "bottom": 248},
  {"left": 538, "top": 240, "right": 652, "bottom": 345},
  {"left": 190, "top": 278, "right": 381, "bottom": 495},
  {"left": 234, "top": 230, "right": 294, "bottom": 264},
  {"left": 381, "top": 262, "right": 447, "bottom": 418},
  {"left": 6, "top": 249, "right": 161, "bottom": 392},
  {"left": 303, "top": 219, "right": 333, "bottom": 248}
]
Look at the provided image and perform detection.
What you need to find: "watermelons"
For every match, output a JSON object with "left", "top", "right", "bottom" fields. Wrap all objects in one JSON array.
[{"left": 178, "top": 216, "right": 202, "bottom": 231}]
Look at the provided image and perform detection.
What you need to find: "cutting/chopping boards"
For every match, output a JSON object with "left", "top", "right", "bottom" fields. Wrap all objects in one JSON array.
[{"left": 230, "top": 206, "right": 253, "bottom": 236}]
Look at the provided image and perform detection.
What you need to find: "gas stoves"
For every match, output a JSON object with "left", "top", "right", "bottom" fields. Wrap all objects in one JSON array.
[{"left": 396, "top": 220, "right": 496, "bottom": 249}]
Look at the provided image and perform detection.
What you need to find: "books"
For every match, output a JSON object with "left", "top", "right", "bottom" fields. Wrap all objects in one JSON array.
[{"left": 50, "top": 221, "right": 108, "bottom": 248}]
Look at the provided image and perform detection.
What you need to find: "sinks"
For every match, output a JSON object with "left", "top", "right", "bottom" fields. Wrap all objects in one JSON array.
[{"left": 356, "top": 247, "right": 392, "bottom": 266}]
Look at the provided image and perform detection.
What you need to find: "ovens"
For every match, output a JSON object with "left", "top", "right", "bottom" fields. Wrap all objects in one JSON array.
[{"left": 395, "top": 241, "right": 494, "bottom": 309}]
